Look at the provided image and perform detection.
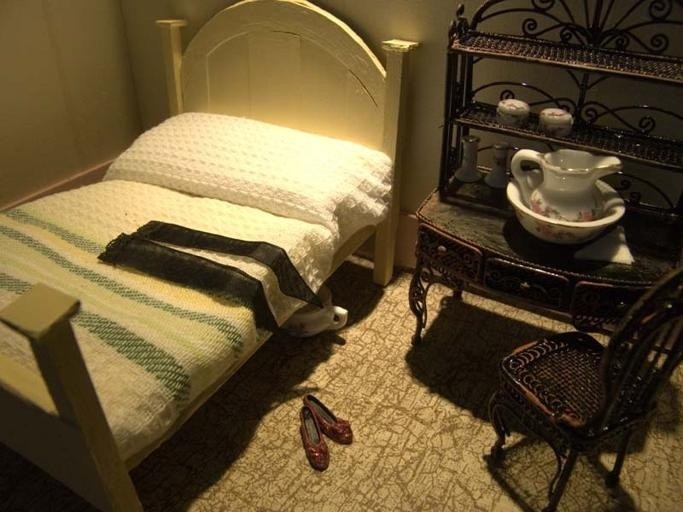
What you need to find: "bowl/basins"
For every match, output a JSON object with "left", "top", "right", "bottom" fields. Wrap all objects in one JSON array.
[{"left": 505, "top": 170, "right": 626, "bottom": 250}]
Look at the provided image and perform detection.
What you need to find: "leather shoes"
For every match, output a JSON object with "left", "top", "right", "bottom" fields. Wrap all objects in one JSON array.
[
  {"left": 300, "top": 406, "right": 329, "bottom": 470},
  {"left": 304, "top": 394, "right": 352, "bottom": 445}
]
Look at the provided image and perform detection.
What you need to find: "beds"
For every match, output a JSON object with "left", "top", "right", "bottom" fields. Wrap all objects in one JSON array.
[{"left": 0, "top": 0, "right": 420, "bottom": 512}]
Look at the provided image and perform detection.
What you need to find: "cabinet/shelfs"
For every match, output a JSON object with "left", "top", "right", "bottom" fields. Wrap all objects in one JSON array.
[{"left": 407, "top": 0, "right": 682, "bottom": 352}]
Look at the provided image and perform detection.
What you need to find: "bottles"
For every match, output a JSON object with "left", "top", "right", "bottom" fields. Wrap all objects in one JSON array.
[
  {"left": 483, "top": 143, "right": 510, "bottom": 188},
  {"left": 453, "top": 134, "right": 483, "bottom": 184}
]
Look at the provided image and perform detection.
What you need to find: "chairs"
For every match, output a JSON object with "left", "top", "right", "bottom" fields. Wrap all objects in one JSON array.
[{"left": 481, "top": 269, "right": 682, "bottom": 511}]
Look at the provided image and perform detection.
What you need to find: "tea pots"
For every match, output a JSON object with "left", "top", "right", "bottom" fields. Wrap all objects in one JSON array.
[{"left": 510, "top": 145, "right": 623, "bottom": 221}]
